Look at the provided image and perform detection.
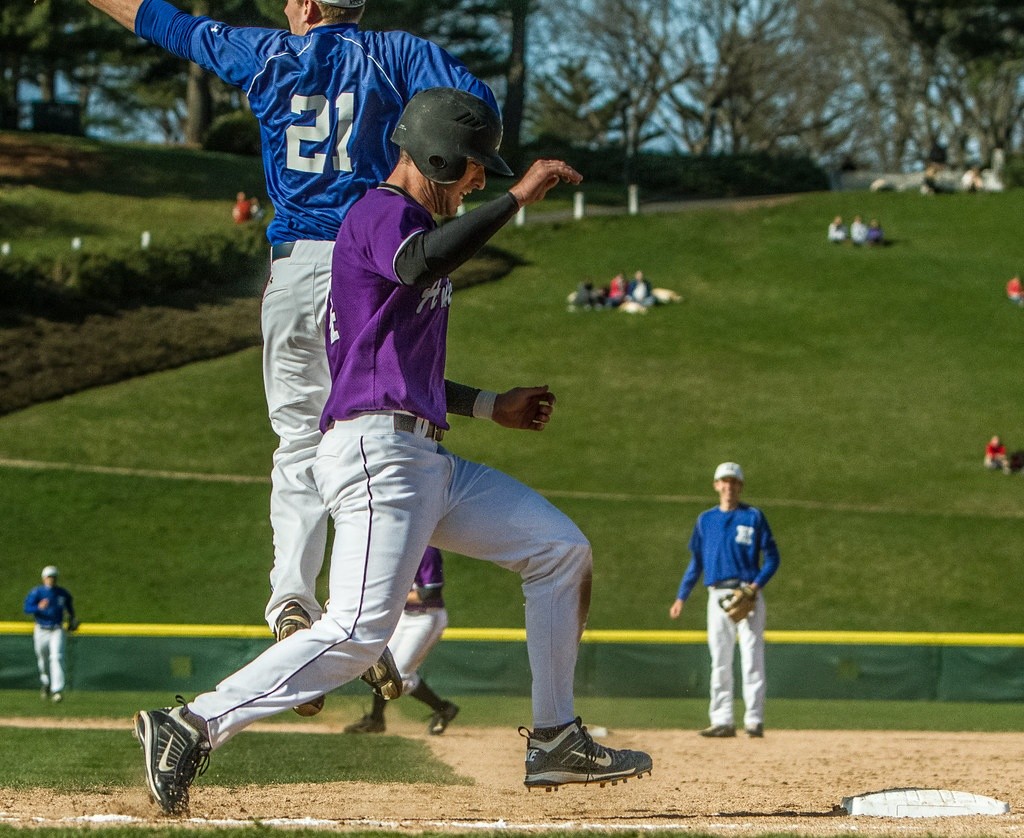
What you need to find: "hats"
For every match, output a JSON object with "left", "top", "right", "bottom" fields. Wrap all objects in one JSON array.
[
  {"left": 714, "top": 462, "right": 745, "bottom": 483},
  {"left": 320, "top": 0, "right": 365, "bottom": 7},
  {"left": 41, "top": 565, "right": 58, "bottom": 579}
]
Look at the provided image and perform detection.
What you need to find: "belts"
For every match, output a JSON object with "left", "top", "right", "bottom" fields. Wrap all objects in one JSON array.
[
  {"left": 41, "top": 626, "right": 56, "bottom": 631},
  {"left": 272, "top": 242, "right": 294, "bottom": 263},
  {"left": 327, "top": 413, "right": 444, "bottom": 442}
]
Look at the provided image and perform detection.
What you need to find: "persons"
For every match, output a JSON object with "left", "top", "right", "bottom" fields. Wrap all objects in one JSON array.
[
  {"left": 232, "top": 191, "right": 265, "bottom": 225},
  {"left": 983, "top": 435, "right": 1011, "bottom": 476},
  {"left": 24, "top": 565, "right": 76, "bottom": 703},
  {"left": 920, "top": 161, "right": 985, "bottom": 195},
  {"left": 827, "top": 213, "right": 883, "bottom": 248},
  {"left": 88, "top": 0, "right": 502, "bottom": 717},
  {"left": 344, "top": 543, "right": 460, "bottom": 736},
  {"left": 568, "top": 270, "right": 684, "bottom": 314},
  {"left": 133, "top": 87, "right": 656, "bottom": 819},
  {"left": 670, "top": 462, "right": 781, "bottom": 739}
]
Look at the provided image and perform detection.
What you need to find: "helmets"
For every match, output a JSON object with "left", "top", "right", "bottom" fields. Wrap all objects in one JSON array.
[{"left": 392, "top": 88, "right": 514, "bottom": 184}]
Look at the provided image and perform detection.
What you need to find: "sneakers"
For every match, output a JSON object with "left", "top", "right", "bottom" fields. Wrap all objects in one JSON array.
[
  {"left": 518, "top": 716, "right": 652, "bottom": 793},
  {"left": 747, "top": 724, "right": 763, "bottom": 737},
  {"left": 428, "top": 700, "right": 461, "bottom": 735},
  {"left": 132, "top": 694, "right": 213, "bottom": 819},
  {"left": 359, "top": 645, "right": 403, "bottom": 701},
  {"left": 697, "top": 724, "right": 736, "bottom": 737},
  {"left": 274, "top": 601, "right": 325, "bottom": 716},
  {"left": 344, "top": 715, "right": 386, "bottom": 735}
]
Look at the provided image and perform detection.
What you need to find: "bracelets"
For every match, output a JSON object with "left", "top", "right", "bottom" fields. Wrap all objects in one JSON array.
[{"left": 472, "top": 389, "right": 497, "bottom": 420}]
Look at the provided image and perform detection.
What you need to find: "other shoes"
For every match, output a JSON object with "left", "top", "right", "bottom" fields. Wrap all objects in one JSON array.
[
  {"left": 52, "top": 693, "right": 62, "bottom": 702},
  {"left": 39, "top": 685, "right": 49, "bottom": 699}
]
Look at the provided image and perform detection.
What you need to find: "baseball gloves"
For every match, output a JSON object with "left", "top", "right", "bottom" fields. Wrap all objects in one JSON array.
[
  {"left": 68, "top": 618, "right": 80, "bottom": 632},
  {"left": 720, "top": 583, "right": 757, "bottom": 624}
]
